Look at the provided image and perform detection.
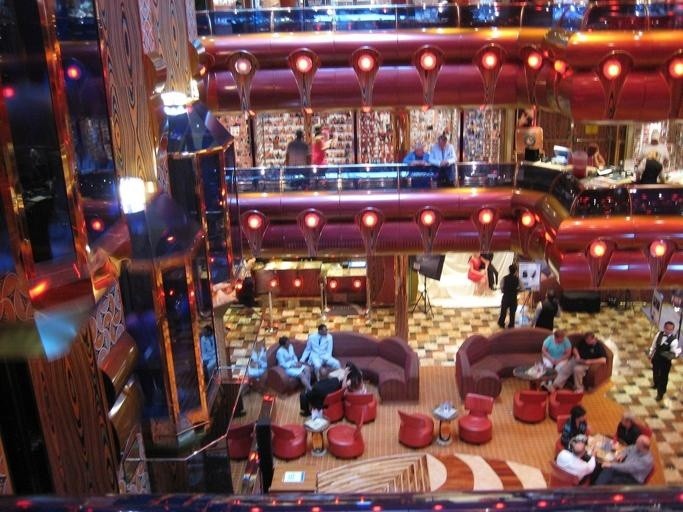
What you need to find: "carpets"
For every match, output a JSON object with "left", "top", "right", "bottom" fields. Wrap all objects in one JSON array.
[{"left": 268, "top": 367, "right": 666, "bottom": 495}]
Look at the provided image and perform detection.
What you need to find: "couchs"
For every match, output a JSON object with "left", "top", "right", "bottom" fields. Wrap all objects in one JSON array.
[
  {"left": 267, "top": 331, "right": 419, "bottom": 401},
  {"left": 455, "top": 328, "right": 614, "bottom": 401}
]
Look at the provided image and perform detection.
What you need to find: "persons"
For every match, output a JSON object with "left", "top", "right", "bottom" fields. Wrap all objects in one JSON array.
[
  {"left": 610, "top": 411, "right": 643, "bottom": 448},
  {"left": 425, "top": 134, "right": 457, "bottom": 186},
  {"left": 593, "top": 433, "right": 655, "bottom": 485},
  {"left": 635, "top": 151, "right": 665, "bottom": 184},
  {"left": 274, "top": 335, "right": 313, "bottom": 394},
  {"left": 284, "top": 127, "right": 310, "bottom": 166},
  {"left": 646, "top": 321, "right": 681, "bottom": 401},
  {"left": 586, "top": 143, "right": 606, "bottom": 171},
  {"left": 311, "top": 135, "right": 328, "bottom": 165},
  {"left": 299, "top": 324, "right": 342, "bottom": 383},
  {"left": 340, "top": 359, "right": 367, "bottom": 395},
  {"left": 636, "top": 139, "right": 670, "bottom": 167},
  {"left": 200, "top": 324, "right": 216, "bottom": 373},
  {"left": 539, "top": 328, "right": 572, "bottom": 392},
  {"left": 542, "top": 331, "right": 606, "bottom": 394},
  {"left": 531, "top": 287, "right": 561, "bottom": 331},
  {"left": 403, "top": 143, "right": 430, "bottom": 188},
  {"left": 479, "top": 252, "right": 498, "bottom": 291},
  {"left": 553, "top": 441, "right": 597, "bottom": 484},
  {"left": 496, "top": 263, "right": 524, "bottom": 328},
  {"left": 465, "top": 251, "right": 490, "bottom": 296},
  {"left": 298, "top": 365, "right": 340, "bottom": 418},
  {"left": 560, "top": 404, "right": 592, "bottom": 462}
]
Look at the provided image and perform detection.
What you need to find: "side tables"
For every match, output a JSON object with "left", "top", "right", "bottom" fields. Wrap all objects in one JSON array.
[
  {"left": 432, "top": 403, "right": 458, "bottom": 446},
  {"left": 304, "top": 414, "right": 331, "bottom": 456}
]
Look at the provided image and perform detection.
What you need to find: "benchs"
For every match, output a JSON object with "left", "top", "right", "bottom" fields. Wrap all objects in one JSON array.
[{"left": 560, "top": 291, "right": 601, "bottom": 313}]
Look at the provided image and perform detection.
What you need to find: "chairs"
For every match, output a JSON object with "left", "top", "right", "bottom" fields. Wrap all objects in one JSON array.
[
  {"left": 271, "top": 424, "right": 307, "bottom": 462},
  {"left": 397, "top": 409, "right": 434, "bottom": 448},
  {"left": 548, "top": 389, "right": 585, "bottom": 421},
  {"left": 458, "top": 392, "right": 493, "bottom": 444},
  {"left": 548, "top": 414, "right": 657, "bottom": 487},
  {"left": 228, "top": 423, "right": 254, "bottom": 462},
  {"left": 310, "top": 387, "right": 344, "bottom": 423},
  {"left": 327, "top": 407, "right": 364, "bottom": 459},
  {"left": 343, "top": 393, "right": 377, "bottom": 422},
  {"left": 514, "top": 389, "right": 548, "bottom": 422}
]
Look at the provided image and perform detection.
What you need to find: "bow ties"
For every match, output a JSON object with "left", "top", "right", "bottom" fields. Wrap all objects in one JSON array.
[{"left": 662, "top": 334, "right": 669, "bottom": 338}]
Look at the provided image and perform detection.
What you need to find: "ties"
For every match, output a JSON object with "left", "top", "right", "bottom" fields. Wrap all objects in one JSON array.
[{"left": 319, "top": 335, "right": 323, "bottom": 344}]
